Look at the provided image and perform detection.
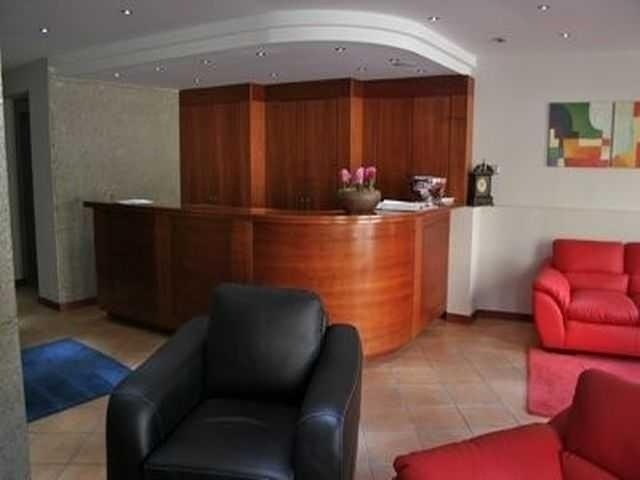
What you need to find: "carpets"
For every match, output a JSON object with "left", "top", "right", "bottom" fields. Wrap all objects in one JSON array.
[
  {"left": 21, "top": 337, "right": 139, "bottom": 426},
  {"left": 525, "top": 345, "right": 640, "bottom": 418}
]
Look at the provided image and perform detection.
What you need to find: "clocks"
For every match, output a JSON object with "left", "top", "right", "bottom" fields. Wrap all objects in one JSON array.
[{"left": 467, "top": 163, "right": 497, "bottom": 207}]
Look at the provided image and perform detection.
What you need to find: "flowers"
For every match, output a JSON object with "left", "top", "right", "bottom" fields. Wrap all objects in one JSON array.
[{"left": 339, "top": 165, "right": 377, "bottom": 190}]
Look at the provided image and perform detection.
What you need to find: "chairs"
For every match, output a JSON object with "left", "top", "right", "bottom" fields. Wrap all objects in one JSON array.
[
  {"left": 105, "top": 283, "right": 363, "bottom": 477},
  {"left": 534, "top": 239, "right": 640, "bottom": 357},
  {"left": 392, "top": 369, "right": 640, "bottom": 479}
]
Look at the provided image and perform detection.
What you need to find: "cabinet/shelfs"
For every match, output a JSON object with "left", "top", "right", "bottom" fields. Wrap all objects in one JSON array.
[
  {"left": 179, "top": 85, "right": 266, "bottom": 208},
  {"left": 267, "top": 79, "right": 364, "bottom": 210},
  {"left": 361, "top": 74, "right": 474, "bottom": 207}
]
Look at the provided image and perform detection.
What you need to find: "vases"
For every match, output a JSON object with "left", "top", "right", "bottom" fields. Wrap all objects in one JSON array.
[{"left": 336, "top": 190, "right": 385, "bottom": 216}]
